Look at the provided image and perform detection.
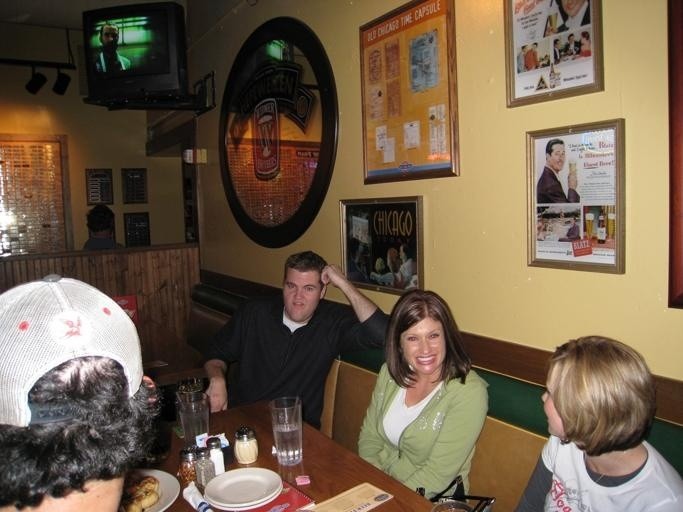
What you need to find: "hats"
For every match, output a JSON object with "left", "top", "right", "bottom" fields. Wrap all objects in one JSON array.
[{"left": 0, "top": 274, "right": 145, "bottom": 429}]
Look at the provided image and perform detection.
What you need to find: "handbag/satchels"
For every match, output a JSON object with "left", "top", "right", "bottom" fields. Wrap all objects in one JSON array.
[{"left": 417, "top": 475, "right": 497, "bottom": 511}]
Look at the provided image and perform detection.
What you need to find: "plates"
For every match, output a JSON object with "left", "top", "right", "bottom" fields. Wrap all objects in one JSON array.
[
  {"left": 205, "top": 466, "right": 281, "bottom": 510},
  {"left": 119, "top": 467, "right": 181, "bottom": 511}
]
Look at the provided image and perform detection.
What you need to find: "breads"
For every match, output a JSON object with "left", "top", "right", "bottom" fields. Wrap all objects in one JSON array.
[{"left": 119, "top": 472, "right": 160, "bottom": 512}]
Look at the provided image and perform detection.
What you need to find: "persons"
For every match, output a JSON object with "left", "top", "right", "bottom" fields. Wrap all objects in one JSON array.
[
  {"left": 524, "top": 42, "right": 540, "bottom": 72},
  {"left": 94, "top": 22, "right": 131, "bottom": 74},
  {"left": 142, "top": 17, "right": 172, "bottom": 71},
  {"left": 512, "top": 335, "right": 682, "bottom": 511},
  {"left": 562, "top": 33, "right": 579, "bottom": 56},
  {"left": 368, "top": 245, "right": 416, "bottom": 286},
  {"left": 554, "top": 0, "right": 590, "bottom": 31},
  {"left": 202, "top": 251, "right": 389, "bottom": 414},
  {"left": 558, "top": 217, "right": 580, "bottom": 242},
  {"left": 0, "top": 274, "right": 158, "bottom": 512},
  {"left": 576, "top": 31, "right": 592, "bottom": 59},
  {"left": 83, "top": 204, "right": 126, "bottom": 252},
  {"left": 516, "top": 43, "right": 527, "bottom": 74},
  {"left": 537, "top": 139, "right": 579, "bottom": 203},
  {"left": 552, "top": 38, "right": 562, "bottom": 65},
  {"left": 357, "top": 289, "right": 487, "bottom": 504}
]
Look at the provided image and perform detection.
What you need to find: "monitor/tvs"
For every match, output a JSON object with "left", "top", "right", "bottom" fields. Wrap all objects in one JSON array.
[{"left": 83, "top": 2, "right": 187, "bottom": 98}]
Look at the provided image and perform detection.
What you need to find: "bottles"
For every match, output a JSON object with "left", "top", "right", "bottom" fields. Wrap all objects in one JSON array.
[
  {"left": 231, "top": 424, "right": 258, "bottom": 466},
  {"left": 597, "top": 209, "right": 606, "bottom": 244},
  {"left": 176, "top": 437, "right": 226, "bottom": 491}
]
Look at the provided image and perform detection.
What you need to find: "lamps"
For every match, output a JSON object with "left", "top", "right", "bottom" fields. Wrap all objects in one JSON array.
[{"left": 0, "top": 28, "right": 77, "bottom": 96}]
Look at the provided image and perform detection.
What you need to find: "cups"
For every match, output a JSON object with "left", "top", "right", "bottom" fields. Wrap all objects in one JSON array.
[
  {"left": 585, "top": 214, "right": 593, "bottom": 240},
  {"left": 173, "top": 388, "right": 207, "bottom": 437},
  {"left": 567, "top": 160, "right": 575, "bottom": 176},
  {"left": 267, "top": 395, "right": 302, "bottom": 467},
  {"left": 176, "top": 374, "right": 205, "bottom": 412},
  {"left": 606, "top": 214, "right": 615, "bottom": 240}
]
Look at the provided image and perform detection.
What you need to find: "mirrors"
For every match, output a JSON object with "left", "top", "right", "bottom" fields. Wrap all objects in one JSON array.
[{"left": 218, "top": 17, "right": 339, "bottom": 248}]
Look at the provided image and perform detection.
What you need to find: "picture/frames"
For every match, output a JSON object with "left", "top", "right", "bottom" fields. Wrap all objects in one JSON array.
[
  {"left": 192, "top": 71, "right": 216, "bottom": 118},
  {"left": 525, "top": 118, "right": 624, "bottom": 274},
  {"left": 503, "top": 1, "right": 604, "bottom": 108},
  {"left": 85, "top": 168, "right": 151, "bottom": 248},
  {"left": 338, "top": 195, "right": 424, "bottom": 297},
  {"left": 358, "top": 0, "right": 461, "bottom": 185}
]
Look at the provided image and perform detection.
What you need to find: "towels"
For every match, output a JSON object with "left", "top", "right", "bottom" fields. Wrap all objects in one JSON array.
[{"left": 182, "top": 480, "right": 214, "bottom": 512}]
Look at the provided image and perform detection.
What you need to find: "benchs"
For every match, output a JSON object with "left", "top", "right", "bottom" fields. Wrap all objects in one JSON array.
[
  {"left": 0, "top": 243, "right": 247, "bottom": 420},
  {"left": 319, "top": 360, "right": 548, "bottom": 512}
]
